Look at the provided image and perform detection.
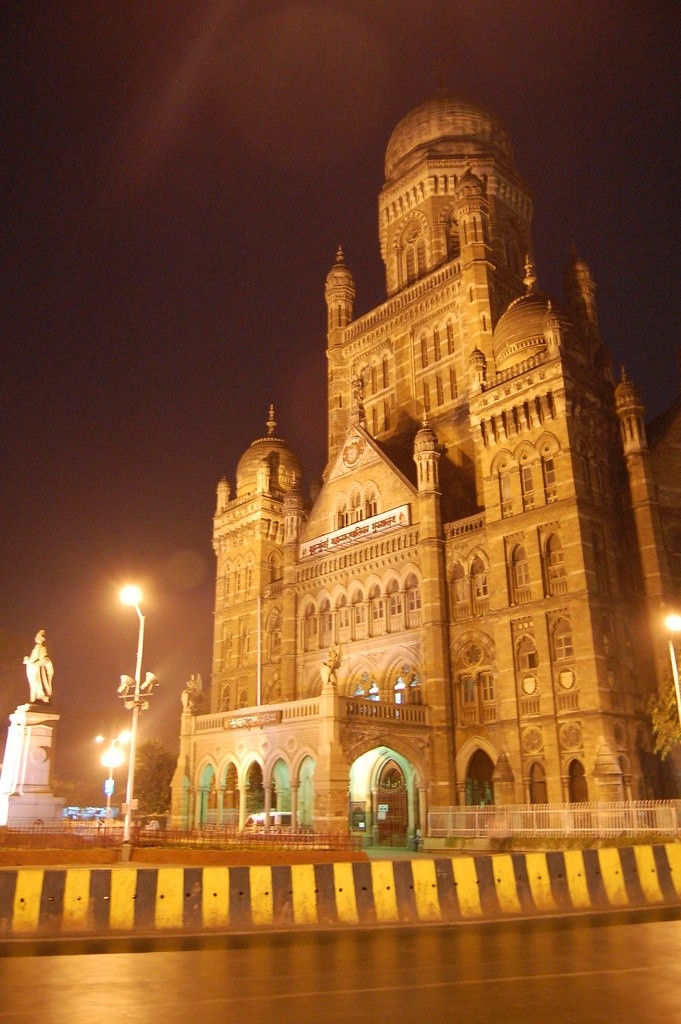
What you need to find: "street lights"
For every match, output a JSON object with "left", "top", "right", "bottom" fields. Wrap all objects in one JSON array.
[
  {"left": 95, "top": 731, "right": 132, "bottom": 845},
  {"left": 663, "top": 613, "right": 681, "bottom": 730},
  {"left": 118, "top": 583, "right": 148, "bottom": 843}
]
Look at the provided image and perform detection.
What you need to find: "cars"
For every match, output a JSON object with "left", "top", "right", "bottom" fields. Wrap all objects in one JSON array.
[{"left": 62, "top": 807, "right": 115, "bottom": 823}]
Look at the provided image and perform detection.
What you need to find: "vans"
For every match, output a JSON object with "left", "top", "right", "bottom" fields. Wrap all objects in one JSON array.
[{"left": 246, "top": 812, "right": 300, "bottom": 834}]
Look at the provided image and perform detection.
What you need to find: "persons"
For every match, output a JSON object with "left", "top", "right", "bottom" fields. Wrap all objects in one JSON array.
[
  {"left": 24, "top": 630, "right": 53, "bottom": 703},
  {"left": 146, "top": 816, "right": 159, "bottom": 831},
  {"left": 413, "top": 824, "right": 421, "bottom": 850}
]
